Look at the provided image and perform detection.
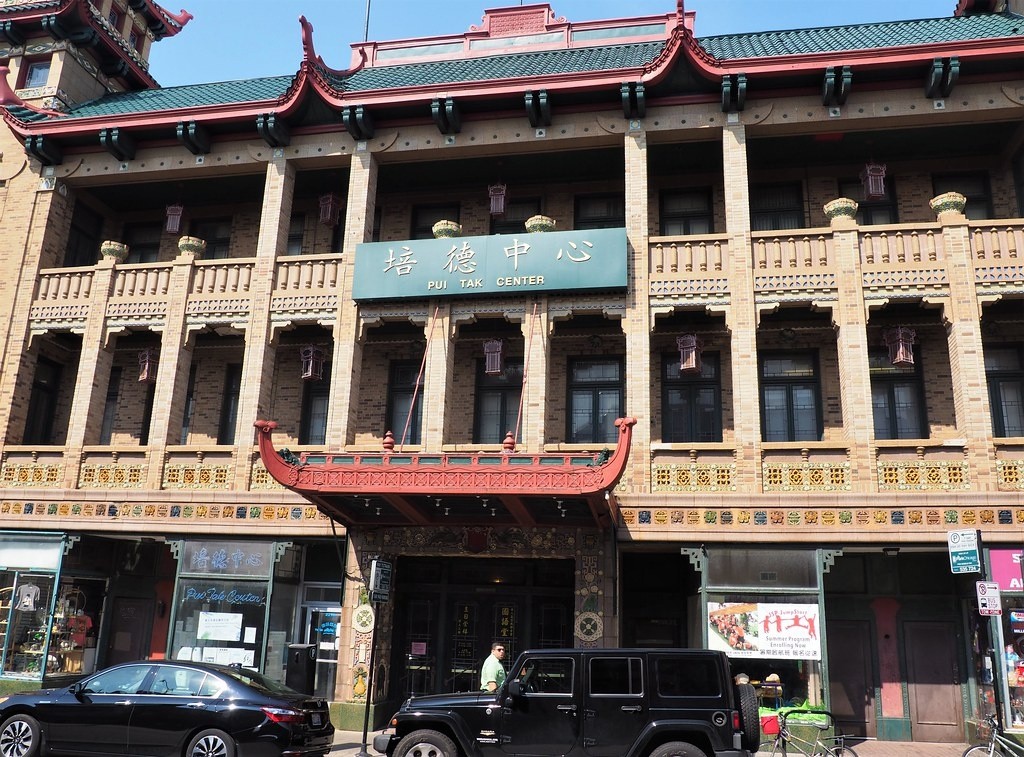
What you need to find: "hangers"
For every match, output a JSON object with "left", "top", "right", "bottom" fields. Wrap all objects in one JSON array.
[{"left": 16, "top": 576, "right": 41, "bottom": 591}]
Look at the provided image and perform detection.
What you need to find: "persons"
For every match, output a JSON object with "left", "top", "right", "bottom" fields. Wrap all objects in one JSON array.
[
  {"left": 479, "top": 642, "right": 506, "bottom": 695},
  {"left": 66, "top": 609, "right": 92, "bottom": 647}
]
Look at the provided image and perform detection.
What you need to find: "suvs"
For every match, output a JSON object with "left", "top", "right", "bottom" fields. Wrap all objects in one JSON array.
[{"left": 373, "top": 647, "right": 762, "bottom": 756}]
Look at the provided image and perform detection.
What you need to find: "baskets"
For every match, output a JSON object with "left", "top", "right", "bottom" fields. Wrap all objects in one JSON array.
[{"left": 760, "top": 713, "right": 779, "bottom": 734}]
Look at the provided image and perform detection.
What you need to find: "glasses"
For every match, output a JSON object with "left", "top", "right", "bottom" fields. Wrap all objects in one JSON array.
[{"left": 494, "top": 649, "right": 504, "bottom": 652}]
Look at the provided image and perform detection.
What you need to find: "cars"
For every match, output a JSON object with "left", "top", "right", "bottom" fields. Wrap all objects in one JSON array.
[{"left": 0, "top": 662, "right": 336, "bottom": 757}]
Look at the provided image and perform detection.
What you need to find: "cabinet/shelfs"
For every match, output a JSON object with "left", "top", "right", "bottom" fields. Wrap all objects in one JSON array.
[{"left": 18, "top": 587, "right": 86, "bottom": 676}]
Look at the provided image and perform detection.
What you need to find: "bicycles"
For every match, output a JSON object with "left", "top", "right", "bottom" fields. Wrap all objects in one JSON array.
[
  {"left": 960, "top": 713, "right": 1024, "bottom": 757},
  {"left": 753, "top": 711, "right": 861, "bottom": 757}
]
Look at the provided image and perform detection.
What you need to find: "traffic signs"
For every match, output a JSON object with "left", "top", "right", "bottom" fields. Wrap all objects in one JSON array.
[{"left": 948, "top": 528, "right": 981, "bottom": 573}]
